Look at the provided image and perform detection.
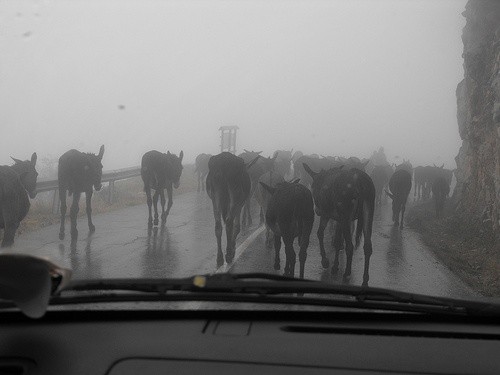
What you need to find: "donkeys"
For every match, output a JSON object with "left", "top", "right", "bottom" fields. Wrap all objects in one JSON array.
[
  {"left": 0, "top": 152, "right": 38, "bottom": 247},
  {"left": 58, "top": 145, "right": 106, "bottom": 239},
  {"left": 140, "top": 150, "right": 184, "bottom": 228},
  {"left": 195, "top": 147, "right": 453, "bottom": 297}
]
